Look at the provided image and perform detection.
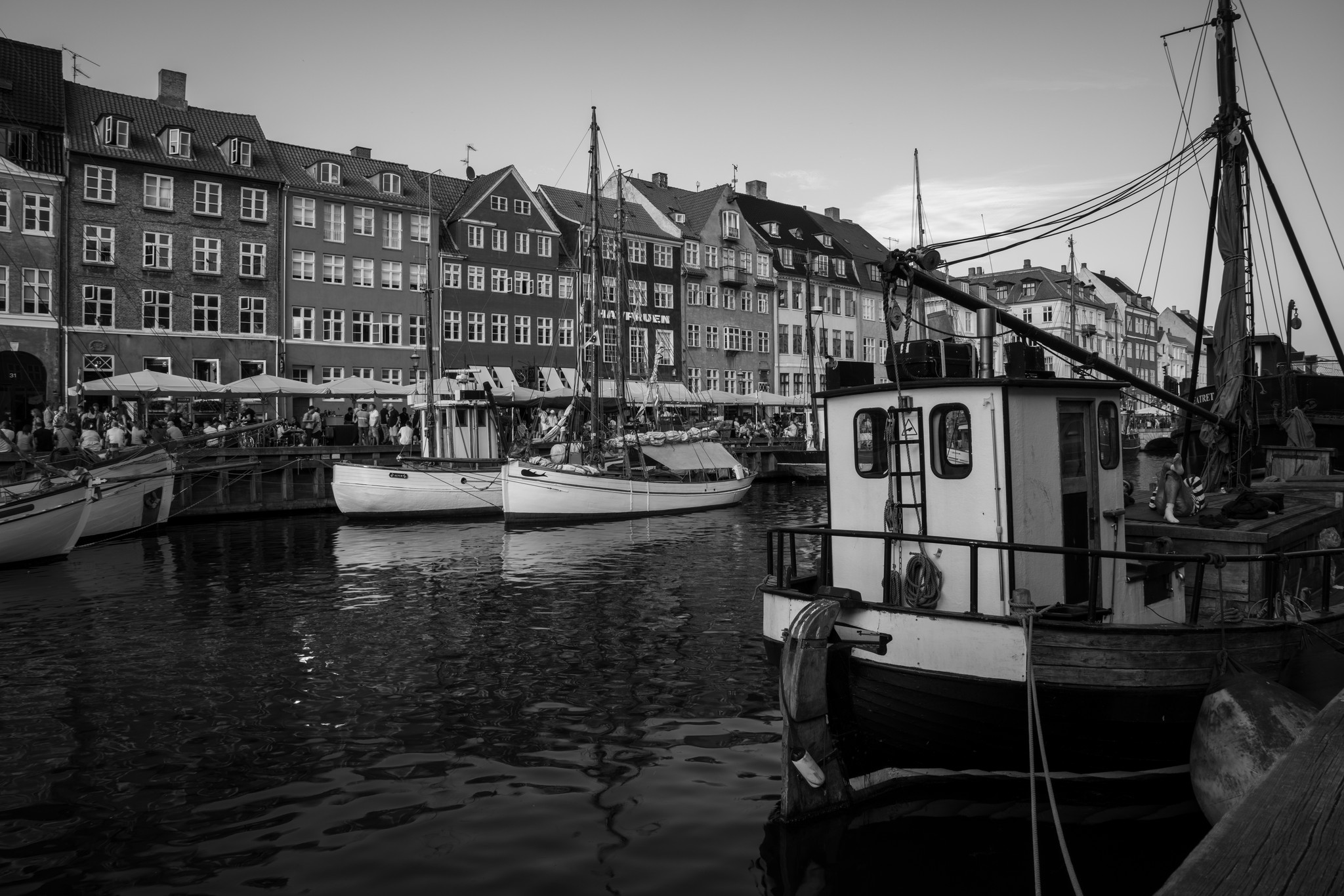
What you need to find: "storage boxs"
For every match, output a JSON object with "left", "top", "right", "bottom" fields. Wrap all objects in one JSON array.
[{"left": 325, "top": 417, "right": 344, "bottom": 425}]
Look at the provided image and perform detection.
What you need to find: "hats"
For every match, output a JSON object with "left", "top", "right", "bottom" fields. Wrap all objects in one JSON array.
[{"left": 550, "top": 410, "right": 555, "bottom": 413}]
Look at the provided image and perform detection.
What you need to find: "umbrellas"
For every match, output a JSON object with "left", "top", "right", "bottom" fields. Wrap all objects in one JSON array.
[
  {"left": 1133, "top": 407, "right": 1166, "bottom": 420},
  {"left": 225, "top": 373, "right": 328, "bottom": 426},
  {"left": 68, "top": 369, "right": 227, "bottom": 431},
  {"left": 635, "top": 386, "right": 712, "bottom": 421},
  {"left": 598, "top": 388, "right": 655, "bottom": 407},
  {"left": 317, "top": 376, "right": 417, "bottom": 414},
  {"left": 403, "top": 369, "right": 610, "bottom": 427},
  {"left": 715, "top": 391, "right": 808, "bottom": 430},
  {"left": 692, "top": 388, "right": 759, "bottom": 421},
  {"left": 788, "top": 392, "right": 824, "bottom": 410},
  {"left": 1120, "top": 407, "right": 1139, "bottom": 415}
]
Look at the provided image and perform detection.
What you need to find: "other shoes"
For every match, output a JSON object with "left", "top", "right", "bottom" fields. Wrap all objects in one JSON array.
[
  {"left": 289, "top": 444, "right": 295, "bottom": 447},
  {"left": 1169, "top": 427, "right": 1173, "bottom": 428},
  {"left": 381, "top": 441, "right": 385, "bottom": 444},
  {"left": 298, "top": 443, "right": 304, "bottom": 446}
]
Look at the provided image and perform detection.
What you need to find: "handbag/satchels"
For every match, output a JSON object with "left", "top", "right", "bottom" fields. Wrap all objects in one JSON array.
[{"left": 538, "top": 423, "right": 544, "bottom": 434}]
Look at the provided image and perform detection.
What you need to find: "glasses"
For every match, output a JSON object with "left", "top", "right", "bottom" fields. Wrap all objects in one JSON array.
[
  {"left": 647, "top": 419, "right": 649, "bottom": 420},
  {"left": 663, "top": 409, "right": 665, "bottom": 410}
]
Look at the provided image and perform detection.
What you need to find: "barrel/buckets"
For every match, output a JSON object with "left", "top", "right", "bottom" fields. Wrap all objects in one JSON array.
[{"left": 550, "top": 442, "right": 585, "bottom": 465}]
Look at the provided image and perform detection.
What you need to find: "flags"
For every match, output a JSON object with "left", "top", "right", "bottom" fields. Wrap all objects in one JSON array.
[
  {"left": 583, "top": 330, "right": 600, "bottom": 348},
  {"left": 76, "top": 370, "right": 84, "bottom": 406}
]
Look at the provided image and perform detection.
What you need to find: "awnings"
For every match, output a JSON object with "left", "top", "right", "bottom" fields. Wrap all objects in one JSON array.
[
  {"left": 539, "top": 367, "right": 564, "bottom": 391},
  {"left": 586, "top": 378, "right": 692, "bottom": 394},
  {"left": 493, "top": 366, "right": 520, "bottom": 388},
  {"left": 561, "top": 368, "right": 588, "bottom": 392},
  {"left": 469, "top": 365, "right": 497, "bottom": 388}
]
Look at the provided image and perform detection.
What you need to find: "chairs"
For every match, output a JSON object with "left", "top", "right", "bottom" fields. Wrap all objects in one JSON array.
[{"left": 267, "top": 427, "right": 288, "bottom": 447}]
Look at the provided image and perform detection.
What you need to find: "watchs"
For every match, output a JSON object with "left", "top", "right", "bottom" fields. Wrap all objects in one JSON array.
[{"left": 120, "top": 425, "right": 123, "bottom": 427}]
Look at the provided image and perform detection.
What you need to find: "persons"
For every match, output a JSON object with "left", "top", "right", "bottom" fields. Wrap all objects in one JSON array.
[
  {"left": 735, "top": 412, "right": 806, "bottom": 447},
  {"left": 1123, "top": 479, "right": 1135, "bottom": 508},
  {"left": 1149, "top": 453, "right": 1205, "bottom": 522},
  {"left": 1130, "top": 410, "right": 1179, "bottom": 428},
  {"left": 0, "top": 400, "right": 421, "bottom": 452},
  {"left": 522, "top": 407, "right": 678, "bottom": 442}
]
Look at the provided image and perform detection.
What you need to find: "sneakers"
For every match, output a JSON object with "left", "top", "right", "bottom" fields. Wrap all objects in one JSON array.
[
  {"left": 1214, "top": 513, "right": 1239, "bottom": 527},
  {"left": 1198, "top": 514, "right": 1222, "bottom": 529}
]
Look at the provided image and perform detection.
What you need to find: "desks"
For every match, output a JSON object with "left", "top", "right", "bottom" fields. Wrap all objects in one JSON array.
[{"left": 290, "top": 430, "right": 301, "bottom": 447}]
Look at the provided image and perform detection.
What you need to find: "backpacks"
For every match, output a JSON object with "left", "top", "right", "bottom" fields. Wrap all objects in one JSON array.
[{"left": 167, "top": 413, "right": 178, "bottom": 422}]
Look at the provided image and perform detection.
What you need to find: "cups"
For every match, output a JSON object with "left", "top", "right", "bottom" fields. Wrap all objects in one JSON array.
[{"left": 641, "top": 421, "right": 643, "bottom": 424}]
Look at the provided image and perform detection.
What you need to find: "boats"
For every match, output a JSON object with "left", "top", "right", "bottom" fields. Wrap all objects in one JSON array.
[
  {"left": 776, "top": 461, "right": 874, "bottom": 480},
  {"left": 0, "top": 442, "right": 176, "bottom": 564},
  {"left": 753, "top": 0, "right": 1344, "bottom": 814}
]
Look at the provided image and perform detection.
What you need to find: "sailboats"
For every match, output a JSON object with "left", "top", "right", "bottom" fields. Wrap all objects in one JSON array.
[
  {"left": 500, "top": 104, "right": 755, "bottom": 530},
  {"left": 329, "top": 235, "right": 523, "bottom": 524}
]
